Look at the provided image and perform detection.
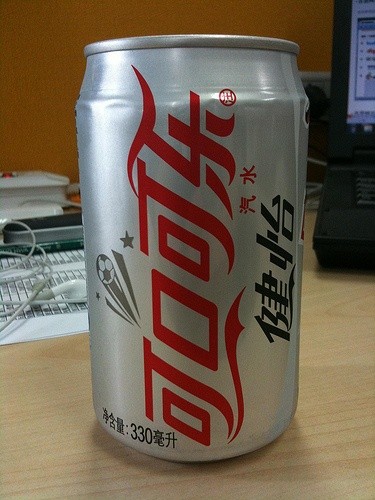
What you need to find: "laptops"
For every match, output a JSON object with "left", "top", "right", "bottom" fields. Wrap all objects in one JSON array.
[{"left": 310, "top": 0, "right": 375, "bottom": 272}]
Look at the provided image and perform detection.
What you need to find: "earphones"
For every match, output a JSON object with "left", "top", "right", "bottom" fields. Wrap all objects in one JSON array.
[{"left": 31, "top": 278, "right": 86, "bottom": 308}]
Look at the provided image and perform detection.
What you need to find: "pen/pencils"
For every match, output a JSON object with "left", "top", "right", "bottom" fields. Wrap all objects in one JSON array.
[
  {"left": 0, "top": 238, "right": 84, "bottom": 251},
  {"left": 0, "top": 241, "right": 85, "bottom": 258}
]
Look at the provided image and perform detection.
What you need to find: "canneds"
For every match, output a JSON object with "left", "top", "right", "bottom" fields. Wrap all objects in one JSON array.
[{"left": 74, "top": 33, "right": 310, "bottom": 463}]
